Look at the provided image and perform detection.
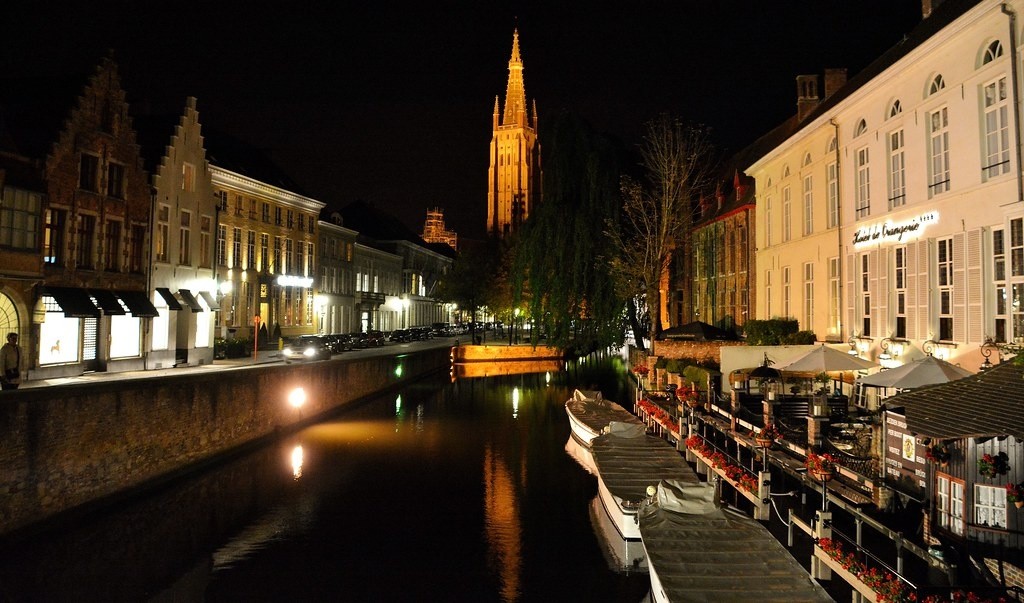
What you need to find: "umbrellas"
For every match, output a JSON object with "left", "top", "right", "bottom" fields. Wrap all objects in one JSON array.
[
  {"left": 779, "top": 344, "right": 879, "bottom": 392},
  {"left": 853, "top": 353, "right": 975, "bottom": 391}
]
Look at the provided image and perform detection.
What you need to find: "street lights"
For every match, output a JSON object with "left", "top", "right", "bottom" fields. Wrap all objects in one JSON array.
[
  {"left": 447, "top": 303, "right": 457, "bottom": 322},
  {"left": 514, "top": 308, "right": 520, "bottom": 345},
  {"left": 530, "top": 319, "right": 535, "bottom": 341}
]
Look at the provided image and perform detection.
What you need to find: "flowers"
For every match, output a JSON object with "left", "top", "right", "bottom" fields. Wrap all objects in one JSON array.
[
  {"left": 632, "top": 365, "right": 649, "bottom": 380},
  {"left": 630, "top": 384, "right": 1020, "bottom": 603}
]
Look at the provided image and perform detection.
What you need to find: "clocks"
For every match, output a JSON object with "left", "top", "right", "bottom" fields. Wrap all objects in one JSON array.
[{"left": 507, "top": 110, "right": 513, "bottom": 117}]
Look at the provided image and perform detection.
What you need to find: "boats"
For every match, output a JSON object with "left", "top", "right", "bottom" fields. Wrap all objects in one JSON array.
[
  {"left": 565, "top": 389, "right": 647, "bottom": 445},
  {"left": 589, "top": 496, "right": 649, "bottom": 575},
  {"left": 637, "top": 475, "right": 836, "bottom": 603},
  {"left": 589, "top": 421, "right": 703, "bottom": 541},
  {"left": 564, "top": 433, "right": 599, "bottom": 477}
]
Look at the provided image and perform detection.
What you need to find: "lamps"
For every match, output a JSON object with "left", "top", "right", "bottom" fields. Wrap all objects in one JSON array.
[
  {"left": 747, "top": 352, "right": 779, "bottom": 378},
  {"left": 848, "top": 335, "right": 1000, "bottom": 372}
]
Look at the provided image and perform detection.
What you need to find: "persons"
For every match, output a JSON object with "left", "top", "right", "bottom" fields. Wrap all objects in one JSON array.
[{"left": 0, "top": 333, "right": 24, "bottom": 388}]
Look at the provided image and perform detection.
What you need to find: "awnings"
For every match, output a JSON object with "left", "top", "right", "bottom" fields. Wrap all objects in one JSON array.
[
  {"left": 179, "top": 289, "right": 203, "bottom": 314},
  {"left": 115, "top": 288, "right": 160, "bottom": 318},
  {"left": 89, "top": 287, "right": 126, "bottom": 316},
  {"left": 49, "top": 285, "right": 102, "bottom": 318},
  {"left": 200, "top": 291, "right": 222, "bottom": 312},
  {"left": 155, "top": 287, "right": 184, "bottom": 312}
]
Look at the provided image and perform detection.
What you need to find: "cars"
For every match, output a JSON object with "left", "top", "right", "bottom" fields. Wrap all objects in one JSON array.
[
  {"left": 282, "top": 335, "right": 331, "bottom": 362},
  {"left": 388, "top": 321, "right": 504, "bottom": 343},
  {"left": 322, "top": 330, "right": 385, "bottom": 355}
]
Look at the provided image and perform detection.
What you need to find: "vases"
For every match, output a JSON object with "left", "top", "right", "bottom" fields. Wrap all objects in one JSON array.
[
  {"left": 812, "top": 471, "right": 831, "bottom": 481},
  {"left": 755, "top": 438, "right": 773, "bottom": 447}
]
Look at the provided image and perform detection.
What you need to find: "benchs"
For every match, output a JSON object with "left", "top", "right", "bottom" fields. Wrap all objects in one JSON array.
[{"left": 738, "top": 391, "right": 864, "bottom": 425}]
